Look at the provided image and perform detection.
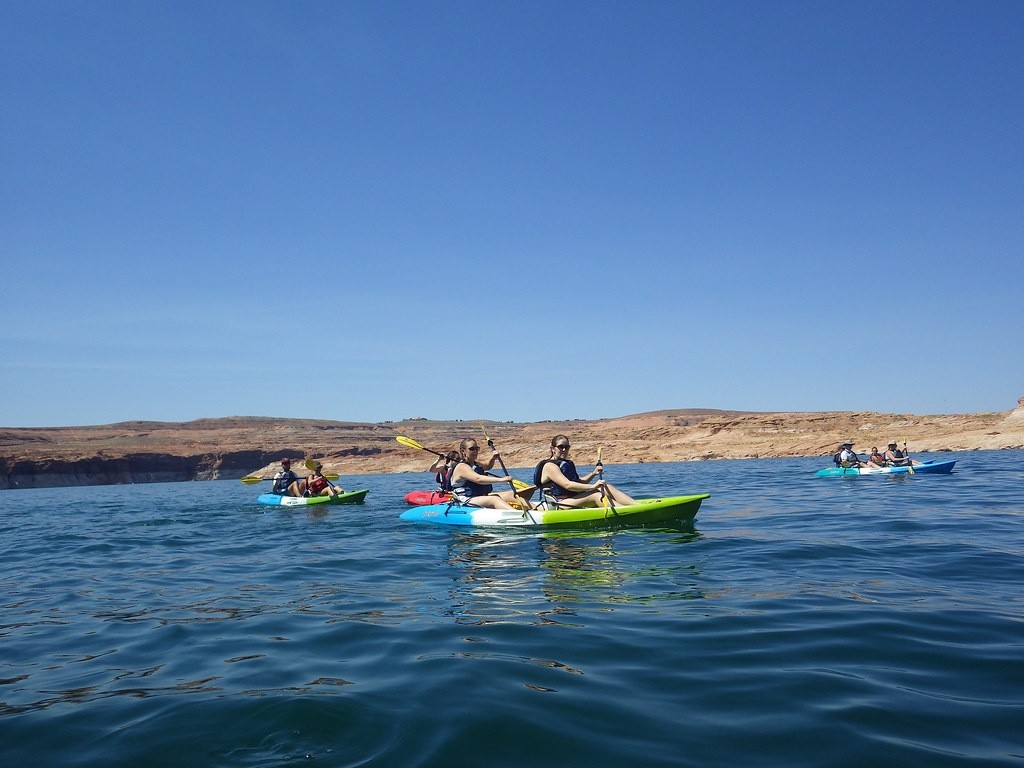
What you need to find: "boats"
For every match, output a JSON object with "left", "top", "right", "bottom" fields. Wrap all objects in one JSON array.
[
  {"left": 403, "top": 490, "right": 455, "bottom": 506},
  {"left": 257, "top": 489, "right": 370, "bottom": 507},
  {"left": 399, "top": 492, "right": 711, "bottom": 528},
  {"left": 813, "top": 459, "right": 960, "bottom": 475}
]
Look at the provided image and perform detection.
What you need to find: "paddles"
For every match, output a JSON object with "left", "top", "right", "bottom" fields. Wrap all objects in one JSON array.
[
  {"left": 903, "top": 438, "right": 915, "bottom": 474},
  {"left": 842, "top": 461, "right": 867, "bottom": 467},
  {"left": 395, "top": 435, "right": 529, "bottom": 489},
  {"left": 240, "top": 472, "right": 339, "bottom": 485},
  {"left": 595, "top": 445, "right": 608, "bottom": 517},
  {"left": 479, "top": 421, "right": 527, "bottom": 519},
  {"left": 305, "top": 459, "right": 336, "bottom": 487}
]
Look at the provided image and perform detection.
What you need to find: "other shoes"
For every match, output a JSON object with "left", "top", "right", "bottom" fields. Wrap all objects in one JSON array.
[
  {"left": 301, "top": 490, "right": 309, "bottom": 497},
  {"left": 337, "top": 490, "right": 344, "bottom": 494}
]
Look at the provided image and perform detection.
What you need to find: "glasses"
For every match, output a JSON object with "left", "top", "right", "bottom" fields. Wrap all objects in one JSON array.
[
  {"left": 553, "top": 444, "right": 570, "bottom": 450},
  {"left": 469, "top": 446, "right": 480, "bottom": 452},
  {"left": 455, "top": 457, "right": 460, "bottom": 461}
]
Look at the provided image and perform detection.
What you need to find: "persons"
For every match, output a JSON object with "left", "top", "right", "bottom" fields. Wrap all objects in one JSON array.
[
  {"left": 884, "top": 441, "right": 922, "bottom": 467},
  {"left": 450, "top": 437, "right": 543, "bottom": 511},
  {"left": 273, "top": 458, "right": 306, "bottom": 497},
  {"left": 429, "top": 451, "right": 459, "bottom": 493},
  {"left": 308, "top": 462, "right": 347, "bottom": 497},
  {"left": 834, "top": 444, "right": 845, "bottom": 468},
  {"left": 534, "top": 435, "right": 661, "bottom": 511},
  {"left": 871, "top": 447, "right": 884, "bottom": 467},
  {"left": 841, "top": 440, "right": 881, "bottom": 468}
]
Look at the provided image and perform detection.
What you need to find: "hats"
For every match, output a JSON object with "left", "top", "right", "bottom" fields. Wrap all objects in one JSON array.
[
  {"left": 838, "top": 445, "right": 845, "bottom": 449},
  {"left": 843, "top": 440, "right": 855, "bottom": 446},
  {"left": 887, "top": 440, "right": 896, "bottom": 446},
  {"left": 314, "top": 461, "right": 323, "bottom": 468},
  {"left": 281, "top": 458, "right": 291, "bottom": 463}
]
